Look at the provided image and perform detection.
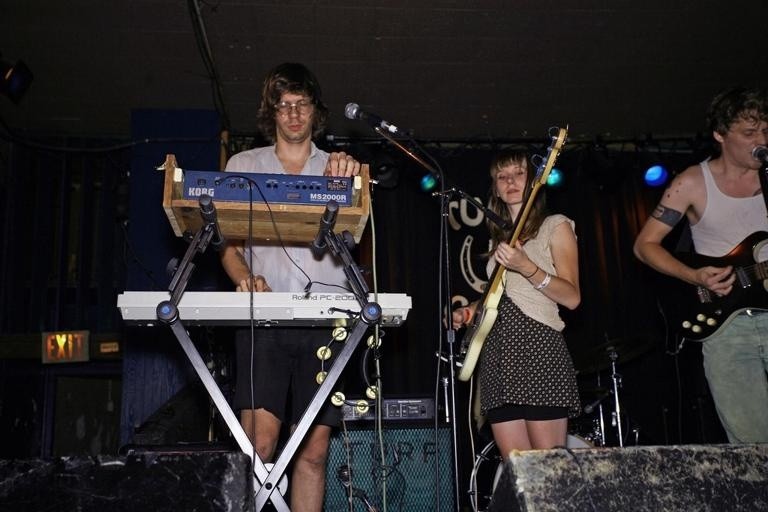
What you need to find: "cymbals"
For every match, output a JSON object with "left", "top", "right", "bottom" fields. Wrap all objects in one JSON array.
[{"left": 571, "top": 331, "right": 649, "bottom": 373}]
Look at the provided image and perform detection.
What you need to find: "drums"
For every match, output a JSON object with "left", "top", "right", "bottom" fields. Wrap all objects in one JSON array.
[
  {"left": 566, "top": 410, "right": 604, "bottom": 448},
  {"left": 467, "top": 432, "right": 596, "bottom": 512}
]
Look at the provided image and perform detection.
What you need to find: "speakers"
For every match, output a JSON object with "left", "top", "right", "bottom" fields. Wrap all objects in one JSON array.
[
  {"left": 484, "top": 442, "right": 768, "bottom": 512},
  {"left": 0, "top": 449, "right": 254, "bottom": 512},
  {"left": 295, "top": 423, "right": 454, "bottom": 512}
]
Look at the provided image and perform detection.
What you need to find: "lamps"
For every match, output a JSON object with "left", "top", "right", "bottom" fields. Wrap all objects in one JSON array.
[
  {"left": 369, "top": 139, "right": 400, "bottom": 187},
  {"left": 638, "top": 139, "right": 668, "bottom": 187}
]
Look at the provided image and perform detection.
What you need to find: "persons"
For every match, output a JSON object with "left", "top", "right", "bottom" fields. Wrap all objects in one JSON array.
[
  {"left": 216, "top": 64, "right": 362, "bottom": 510},
  {"left": 631, "top": 79, "right": 768, "bottom": 445},
  {"left": 442, "top": 132, "right": 585, "bottom": 464}
]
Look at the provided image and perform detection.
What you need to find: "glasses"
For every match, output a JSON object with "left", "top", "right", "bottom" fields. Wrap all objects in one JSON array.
[{"left": 273, "top": 99, "right": 313, "bottom": 115}]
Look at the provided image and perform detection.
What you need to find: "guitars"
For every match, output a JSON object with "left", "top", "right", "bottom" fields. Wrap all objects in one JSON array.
[
  {"left": 454, "top": 125, "right": 570, "bottom": 382},
  {"left": 653, "top": 231, "right": 768, "bottom": 342}
]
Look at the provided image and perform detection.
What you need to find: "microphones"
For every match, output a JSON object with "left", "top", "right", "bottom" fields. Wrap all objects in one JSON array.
[
  {"left": 751, "top": 145, "right": 768, "bottom": 174},
  {"left": 343, "top": 103, "right": 398, "bottom": 137}
]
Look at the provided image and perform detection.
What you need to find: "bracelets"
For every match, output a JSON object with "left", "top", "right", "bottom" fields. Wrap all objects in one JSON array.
[
  {"left": 533, "top": 273, "right": 553, "bottom": 293},
  {"left": 523, "top": 267, "right": 540, "bottom": 280},
  {"left": 461, "top": 305, "right": 471, "bottom": 324}
]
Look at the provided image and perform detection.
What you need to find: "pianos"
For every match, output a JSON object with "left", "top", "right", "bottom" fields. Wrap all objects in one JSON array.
[{"left": 116, "top": 291, "right": 412, "bottom": 328}]
